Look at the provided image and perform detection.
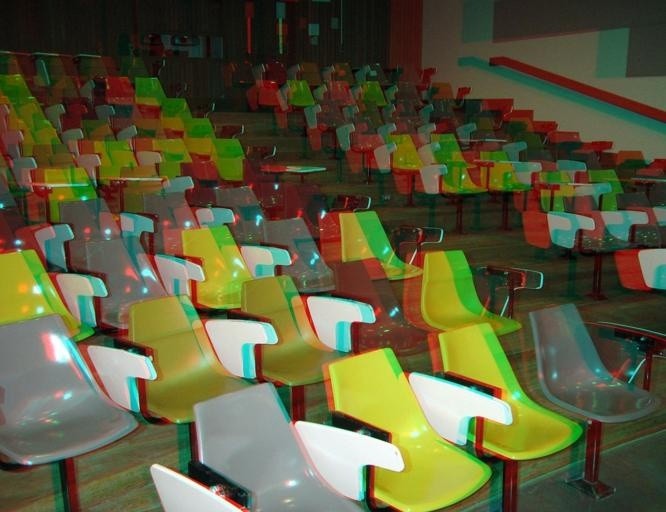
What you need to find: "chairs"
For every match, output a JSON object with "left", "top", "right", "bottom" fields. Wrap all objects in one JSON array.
[
  {"left": 148, "top": 301, "right": 665, "bottom": 512},
  {"left": 1, "top": 2, "right": 522, "bottom": 460},
  {"left": 231, "top": 57, "right": 665, "bottom": 298}
]
